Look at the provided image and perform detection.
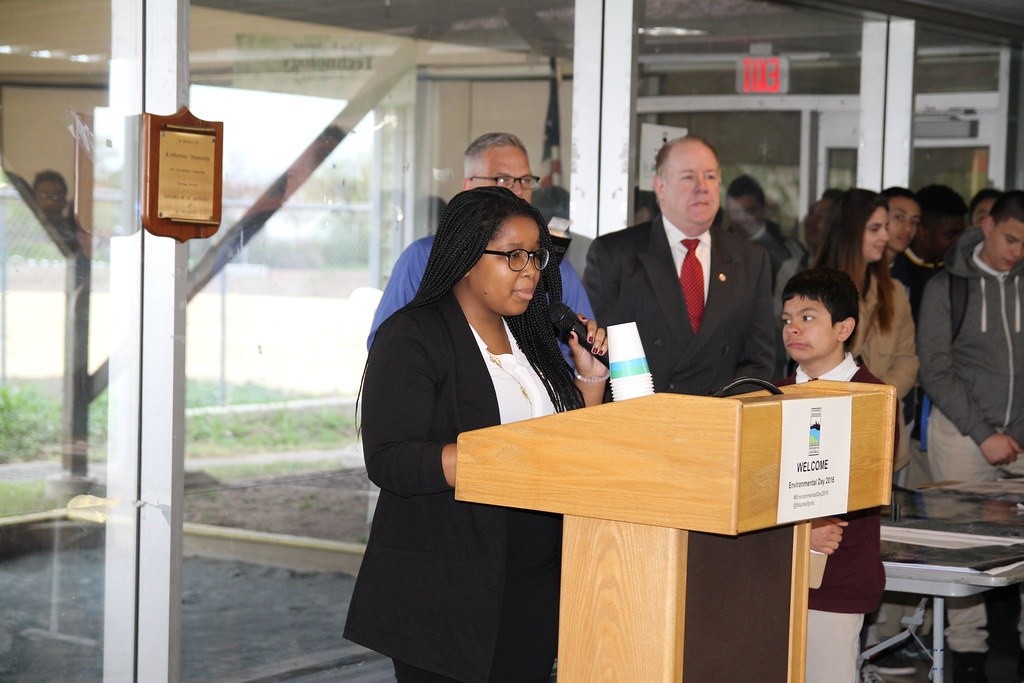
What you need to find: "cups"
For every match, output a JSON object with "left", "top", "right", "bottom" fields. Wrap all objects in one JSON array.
[{"left": 606, "top": 322, "right": 654, "bottom": 401}]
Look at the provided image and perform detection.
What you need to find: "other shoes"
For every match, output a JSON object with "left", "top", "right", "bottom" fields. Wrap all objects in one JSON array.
[
  {"left": 899, "top": 639, "right": 931, "bottom": 657},
  {"left": 952, "top": 651, "right": 989, "bottom": 683},
  {"left": 861, "top": 644, "right": 917, "bottom": 674}
]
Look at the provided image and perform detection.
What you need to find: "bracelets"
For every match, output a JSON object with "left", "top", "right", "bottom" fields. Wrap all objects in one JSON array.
[{"left": 573, "top": 369, "right": 610, "bottom": 382}]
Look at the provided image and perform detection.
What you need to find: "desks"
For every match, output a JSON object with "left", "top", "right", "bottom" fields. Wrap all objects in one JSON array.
[{"left": 859, "top": 455, "right": 1024, "bottom": 683}]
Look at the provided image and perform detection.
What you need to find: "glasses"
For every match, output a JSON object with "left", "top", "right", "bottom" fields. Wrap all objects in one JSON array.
[
  {"left": 470, "top": 175, "right": 540, "bottom": 191},
  {"left": 39, "top": 192, "right": 62, "bottom": 201},
  {"left": 483, "top": 249, "right": 552, "bottom": 271}
]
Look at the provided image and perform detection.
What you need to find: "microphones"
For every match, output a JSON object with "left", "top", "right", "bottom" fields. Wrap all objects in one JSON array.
[{"left": 548, "top": 302, "right": 609, "bottom": 369}]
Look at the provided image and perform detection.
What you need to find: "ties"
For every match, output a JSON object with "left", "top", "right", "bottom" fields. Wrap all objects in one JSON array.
[{"left": 680, "top": 239, "right": 704, "bottom": 338}]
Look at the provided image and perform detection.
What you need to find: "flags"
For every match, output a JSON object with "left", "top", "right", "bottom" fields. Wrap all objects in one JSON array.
[{"left": 537, "top": 77, "right": 563, "bottom": 186}]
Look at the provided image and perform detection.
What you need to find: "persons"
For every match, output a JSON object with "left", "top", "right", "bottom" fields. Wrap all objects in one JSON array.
[
  {"left": 418, "top": 196, "right": 447, "bottom": 235},
  {"left": 801, "top": 185, "right": 1024, "bottom": 683},
  {"left": 531, "top": 185, "right": 569, "bottom": 226},
  {"left": 342, "top": 188, "right": 612, "bottom": 683},
  {"left": 581, "top": 135, "right": 775, "bottom": 398},
  {"left": 633, "top": 189, "right": 660, "bottom": 225},
  {"left": 725, "top": 173, "right": 802, "bottom": 295},
  {"left": 33, "top": 171, "right": 80, "bottom": 252},
  {"left": 366, "top": 133, "right": 600, "bottom": 371},
  {"left": 778, "top": 268, "right": 901, "bottom": 683}
]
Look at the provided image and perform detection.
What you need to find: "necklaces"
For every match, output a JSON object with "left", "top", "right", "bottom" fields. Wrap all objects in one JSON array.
[{"left": 488, "top": 351, "right": 529, "bottom": 398}]
